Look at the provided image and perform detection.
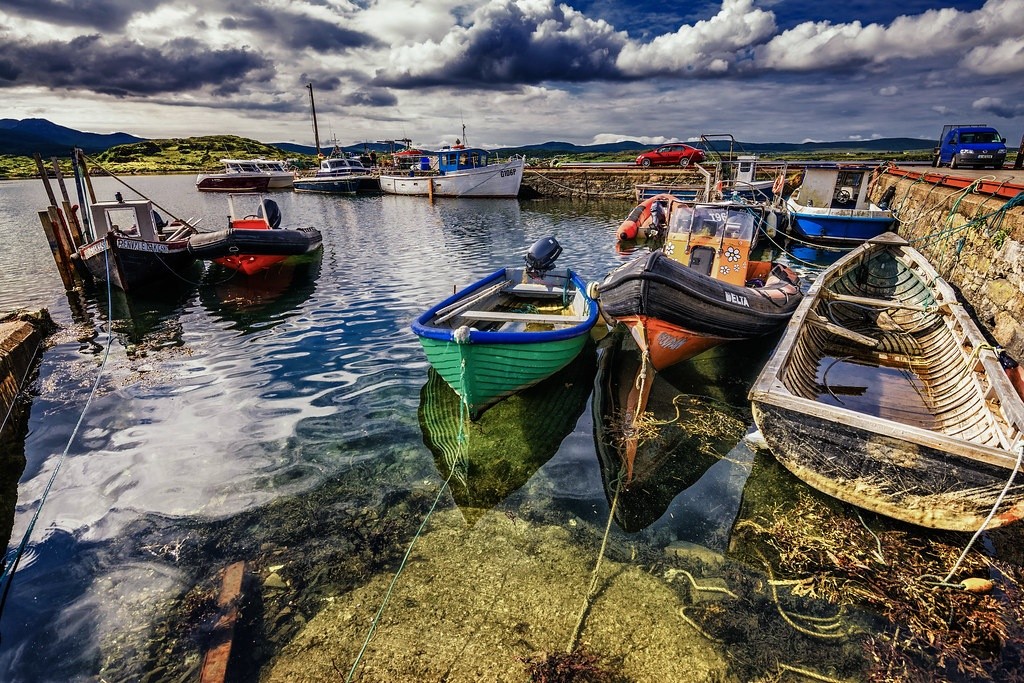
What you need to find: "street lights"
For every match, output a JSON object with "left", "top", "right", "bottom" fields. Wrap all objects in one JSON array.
[{"left": 306, "top": 82, "right": 321, "bottom": 169}]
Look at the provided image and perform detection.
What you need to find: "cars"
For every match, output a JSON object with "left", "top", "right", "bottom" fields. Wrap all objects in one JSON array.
[
  {"left": 936, "top": 127, "right": 1007, "bottom": 169},
  {"left": 636, "top": 144, "right": 706, "bottom": 167}
]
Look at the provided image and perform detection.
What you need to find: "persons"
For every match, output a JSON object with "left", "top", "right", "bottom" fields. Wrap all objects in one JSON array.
[
  {"left": 460, "top": 153, "right": 467, "bottom": 165},
  {"left": 370, "top": 150, "right": 376, "bottom": 167},
  {"left": 331, "top": 145, "right": 342, "bottom": 158},
  {"left": 318, "top": 151, "right": 326, "bottom": 169}
]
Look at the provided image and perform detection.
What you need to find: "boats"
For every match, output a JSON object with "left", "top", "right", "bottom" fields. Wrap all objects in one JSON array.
[
  {"left": 76, "top": 193, "right": 199, "bottom": 293},
  {"left": 417, "top": 339, "right": 599, "bottom": 527},
  {"left": 198, "top": 243, "right": 323, "bottom": 332},
  {"left": 188, "top": 193, "right": 323, "bottom": 275},
  {"left": 616, "top": 194, "right": 690, "bottom": 242},
  {"left": 379, "top": 145, "right": 526, "bottom": 199},
  {"left": 747, "top": 231, "right": 1024, "bottom": 532},
  {"left": 595, "top": 199, "right": 804, "bottom": 373},
  {"left": 786, "top": 164, "right": 899, "bottom": 244},
  {"left": 293, "top": 158, "right": 380, "bottom": 193},
  {"left": 635, "top": 134, "right": 790, "bottom": 246},
  {"left": 411, "top": 266, "right": 600, "bottom": 418},
  {"left": 195, "top": 172, "right": 271, "bottom": 193},
  {"left": 219, "top": 158, "right": 295, "bottom": 188},
  {"left": 594, "top": 321, "right": 787, "bottom": 532}
]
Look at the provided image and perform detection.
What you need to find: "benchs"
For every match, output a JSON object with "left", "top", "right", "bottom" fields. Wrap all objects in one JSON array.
[
  {"left": 461, "top": 311, "right": 589, "bottom": 325},
  {"left": 502, "top": 283, "right": 576, "bottom": 296},
  {"left": 162, "top": 226, "right": 186, "bottom": 232},
  {"left": 820, "top": 285, "right": 951, "bottom": 316}
]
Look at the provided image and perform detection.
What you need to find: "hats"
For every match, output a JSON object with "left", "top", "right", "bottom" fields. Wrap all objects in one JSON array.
[{"left": 372, "top": 150, "right": 375, "bottom": 153}]
